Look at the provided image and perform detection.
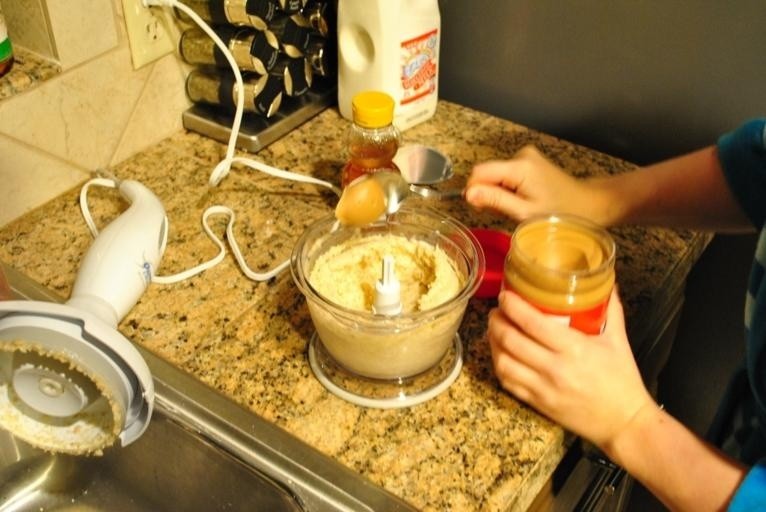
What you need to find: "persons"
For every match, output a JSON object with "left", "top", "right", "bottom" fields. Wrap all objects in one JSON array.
[{"left": 460, "top": 115, "right": 766, "bottom": 512}]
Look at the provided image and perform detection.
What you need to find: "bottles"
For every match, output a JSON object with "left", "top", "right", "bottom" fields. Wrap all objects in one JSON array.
[{"left": 338, "top": 89, "right": 402, "bottom": 194}]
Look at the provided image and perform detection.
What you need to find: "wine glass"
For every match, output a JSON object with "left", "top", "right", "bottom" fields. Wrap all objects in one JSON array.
[{"left": 287, "top": 200, "right": 488, "bottom": 411}]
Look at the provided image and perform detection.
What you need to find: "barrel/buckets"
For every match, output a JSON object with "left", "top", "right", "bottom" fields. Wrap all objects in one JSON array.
[{"left": 336, "top": 1, "right": 440, "bottom": 133}]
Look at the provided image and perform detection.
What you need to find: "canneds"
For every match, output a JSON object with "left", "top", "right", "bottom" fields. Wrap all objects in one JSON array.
[{"left": 0, "top": 7, "right": 16, "bottom": 76}]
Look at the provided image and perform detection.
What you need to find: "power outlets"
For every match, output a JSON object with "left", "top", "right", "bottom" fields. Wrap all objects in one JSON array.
[{"left": 122, "top": 0, "right": 175, "bottom": 69}]
